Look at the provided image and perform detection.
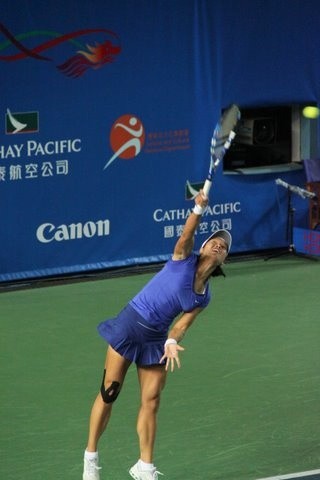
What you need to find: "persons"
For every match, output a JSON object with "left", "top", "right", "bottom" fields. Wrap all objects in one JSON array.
[{"left": 82, "top": 192, "right": 232, "bottom": 480}]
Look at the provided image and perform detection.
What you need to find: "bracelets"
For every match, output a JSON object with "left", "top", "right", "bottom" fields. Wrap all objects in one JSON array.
[
  {"left": 164, "top": 338, "right": 177, "bottom": 348},
  {"left": 192, "top": 205, "right": 204, "bottom": 215}
]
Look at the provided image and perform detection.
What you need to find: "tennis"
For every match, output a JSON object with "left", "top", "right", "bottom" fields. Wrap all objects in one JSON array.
[{"left": 302, "top": 106, "right": 320, "bottom": 119}]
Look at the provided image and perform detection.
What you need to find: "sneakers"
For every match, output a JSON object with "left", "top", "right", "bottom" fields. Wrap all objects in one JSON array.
[
  {"left": 82, "top": 448, "right": 102, "bottom": 480},
  {"left": 128, "top": 460, "right": 164, "bottom": 480}
]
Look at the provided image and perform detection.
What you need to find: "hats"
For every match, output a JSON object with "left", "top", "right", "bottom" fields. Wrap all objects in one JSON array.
[{"left": 199, "top": 229, "right": 232, "bottom": 252}]
